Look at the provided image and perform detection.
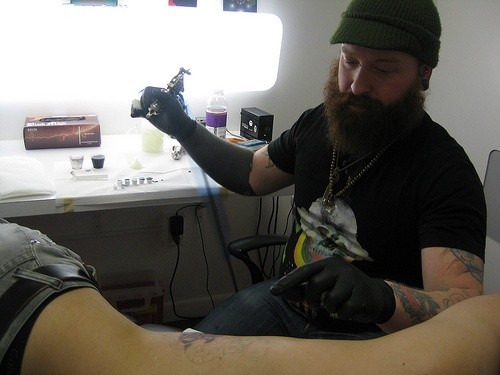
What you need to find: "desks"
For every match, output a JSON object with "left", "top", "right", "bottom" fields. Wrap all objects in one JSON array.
[{"left": 0, "top": 135, "right": 296, "bottom": 222}]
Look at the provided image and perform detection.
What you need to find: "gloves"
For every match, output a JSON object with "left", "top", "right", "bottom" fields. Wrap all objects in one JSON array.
[
  {"left": 271, "top": 255, "right": 397, "bottom": 325},
  {"left": 130, "top": 86, "right": 197, "bottom": 142}
]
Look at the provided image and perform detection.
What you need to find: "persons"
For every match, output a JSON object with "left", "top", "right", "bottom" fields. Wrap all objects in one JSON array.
[{"left": 0, "top": 0, "right": 500, "bottom": 375}]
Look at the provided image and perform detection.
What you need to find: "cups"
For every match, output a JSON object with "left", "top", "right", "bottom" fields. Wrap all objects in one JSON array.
[
  {"left": 71, "top": 155, "right": 84, "bottom": 169},
  {"left": 92, "top": 155, "right": 106, "bottom": 169}
]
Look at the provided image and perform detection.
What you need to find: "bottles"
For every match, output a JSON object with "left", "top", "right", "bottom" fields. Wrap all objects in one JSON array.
[{"left": 206, "top": 90, "right": 229, "bottom": 139}]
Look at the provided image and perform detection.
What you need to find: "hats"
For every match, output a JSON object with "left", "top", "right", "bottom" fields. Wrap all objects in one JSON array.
[{"left": 330, "top": 0, "right": 441, "bottom": 69}]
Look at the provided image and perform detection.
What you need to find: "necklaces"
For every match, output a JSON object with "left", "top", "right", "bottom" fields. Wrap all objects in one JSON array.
[
  {"left": 332, "top": 140, "right": 391, "bottom": 186},
  {"left": 325, "top": 134, "right": 400, "bottom": 206}
]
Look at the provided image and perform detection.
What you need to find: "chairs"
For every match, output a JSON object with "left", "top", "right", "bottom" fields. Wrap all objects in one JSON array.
[{"left": 227, "top": 149, "right": 500, "bottom": 339}]
